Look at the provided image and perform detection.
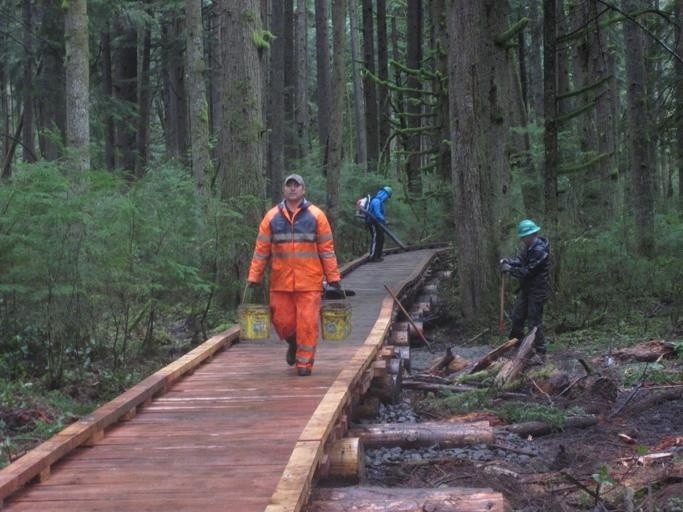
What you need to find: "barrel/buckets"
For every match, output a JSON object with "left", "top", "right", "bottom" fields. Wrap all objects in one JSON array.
[
  {"left": 237, "top": 283, "right": 271, "bottom": 344},
  {"left": 319, "top": 282, "right": 352, "bottom": 342}
]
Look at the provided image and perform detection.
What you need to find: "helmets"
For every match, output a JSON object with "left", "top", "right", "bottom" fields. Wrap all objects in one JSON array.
[
  {"left": 381, "top": 187, "right": 392, "bottom": 197},
  {"left": 517, "top": 220, "right": 540, "bottom": 238}
]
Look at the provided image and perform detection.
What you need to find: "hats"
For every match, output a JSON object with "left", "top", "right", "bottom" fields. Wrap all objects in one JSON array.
[{"left": 284, "top": 174, "right": 304, "bottom": 185}]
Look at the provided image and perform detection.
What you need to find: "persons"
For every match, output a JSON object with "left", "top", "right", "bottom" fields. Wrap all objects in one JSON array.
[
  {"left": 248, "top": 173, "right": 341, "bottom": 379},
  {"left": 499, "top": 220, "right": 550, "bottom": 352},
  {"left": 368, "top": 186, "right": 394, "bottom": 262}
]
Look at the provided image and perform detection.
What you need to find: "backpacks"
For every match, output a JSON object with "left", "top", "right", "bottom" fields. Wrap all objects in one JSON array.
[{"left": 355, "top": 194, "right": 376, "bottom": 222}]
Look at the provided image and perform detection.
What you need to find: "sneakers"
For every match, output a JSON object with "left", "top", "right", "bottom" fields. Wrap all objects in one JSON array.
[
  {"left": 284, "top": 334, "right": 296, "bottom": 364},
  {"left": 298, "top": 367, "right": 311, "bottom": 376},
  {"left": 368, "top": 256, "right": 383, "bottom": 262}
]
